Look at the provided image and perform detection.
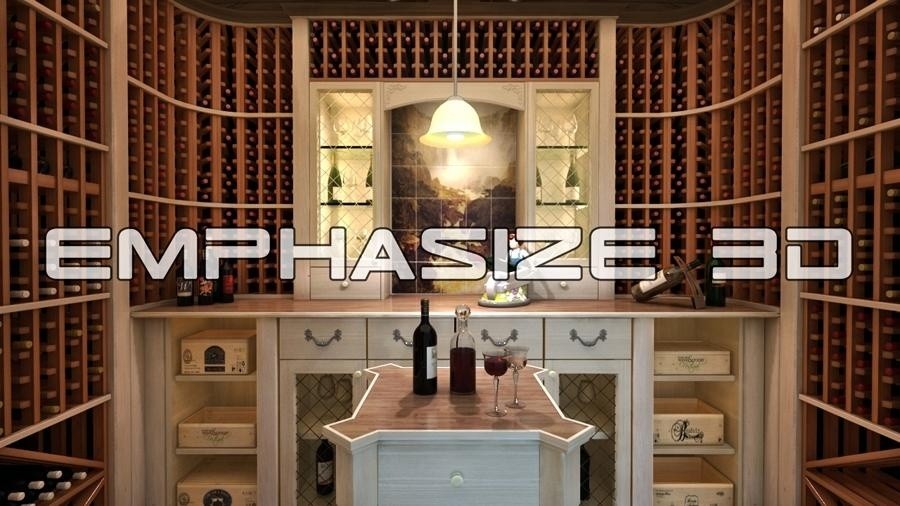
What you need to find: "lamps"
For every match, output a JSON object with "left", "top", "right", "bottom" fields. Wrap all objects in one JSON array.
[{"left": 419, "top": 0, "right": 489, "bottom": 152}]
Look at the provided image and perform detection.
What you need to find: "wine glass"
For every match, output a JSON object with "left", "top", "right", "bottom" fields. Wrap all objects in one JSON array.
[
  {"left": 317, "top": 375, "right": 352, "bottom": 420},
  {"left": 346, "top": 227, "right": 370, "bottom": 259},
  {"left": 483, "top": 348, "right": 512, "bottom": 417},
  {"left": 560, "top": 113, "right": 578, "bottom": 147},
  {"left": 343, "top": 174, "right": 360, "bottom": 203},
  {"left": 538, "top": 115, "right": 553, "bottom": 145},
  {"left": 504, "top": 347, "right": 531, "bottom": 409},
  {"left": 333, "top": 115, "right": 372, "bottom": 145},
  {"left": 577, "top": 380, "right": 597, "bottom": 428}
]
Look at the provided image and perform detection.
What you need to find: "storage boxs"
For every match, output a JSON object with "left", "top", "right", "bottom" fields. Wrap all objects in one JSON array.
[
  {"left": 176, "top": 461, "right": 257, "bottom": 506},
  {"left": 656, "top": 398, "right": 724, "bottom": 445},
  {"left": 652, "top": 456, "right": 736, "bottom": 506},
  {"left": 179, "top": 405, "right": 254, "bottom": 449},
  {"left": 181, "top": 328, "right": 254, "bottom": 374},
  {"left": 656, "top": 340, "right": 730, "bottom": 374}
]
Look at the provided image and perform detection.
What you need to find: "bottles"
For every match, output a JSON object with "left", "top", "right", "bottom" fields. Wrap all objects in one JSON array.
[
  {"left": 411, "top": 298, "right": 438, "bottom": 394},
  {"left": 564, "top": 152, "right": 580, "bottom": 204},
  {"left": 450, "top": 303, "right": 475, "bottom": 396},
  {"left": 632, "top": 256, "right": 704, "bottom": 301},
  {"left": 535, "top": 166, "right": 542, "bottom": 204},
  {"left": 0, "top": 467, "right": 87, "bottom": 505},
  {"left": 580, "top": 443, "right": 592, "bottom": 501},
  {"left": 175, "top": 252, "right": 235, "bottom": 306},
  {"left": 366, "top": 150, "right": 373, "bottom": 203},
  {"left": 328, "top": 152, "right": 341, "bottom": 203},
  {"left": 317, "top": 439, "right": 334, "bottom": 497},
  {"left": 706, "top": 240, "right": 725, "bottom": 306}
]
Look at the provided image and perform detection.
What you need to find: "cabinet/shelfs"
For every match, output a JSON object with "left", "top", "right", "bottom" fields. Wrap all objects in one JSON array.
[
  {"left": 312, "top": 84, "right": 387, "bottom": 298},
  {"left": 459, "top": 318, "right": 633, "bottom": 505},
  {"left": 318, "top": 362, "right": 599, "bottom": 506},
  {"left": 279, "top": 320, "right": 457, "bottom": 506},
  {"left": 129, "top": 1, "right": 295, "bottom": 506},
  {"left": 521, "top": 83, "right": 601, "bottom": 302},
  {"left": 616, "top": 2, "right": 774, "bottom": 505},
  {"left": 774, "top": 1, "right": 900, "bottom": 506},
  {"left": 139, "top": 308, "right": 276, "bottom": 506},
  {"left": 633, "top": 318, "right": 770, "bottom": 506},
  {"left": 2, "top": 0, "right": 131, "bottom": 506},
  {"left": 310, "top": 17, "right": 598, "bottom": 81}
]
